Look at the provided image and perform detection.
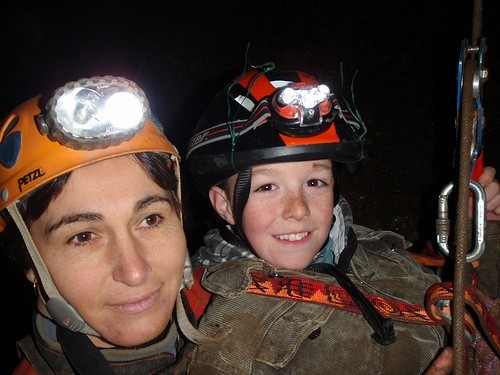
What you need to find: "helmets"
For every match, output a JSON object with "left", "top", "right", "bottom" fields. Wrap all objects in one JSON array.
[
  {"left": 0, "top": 73, "right": 180, "bottom": 210},
  {"left": 185, "top": 62, "right": 363, "bottom": 195}
]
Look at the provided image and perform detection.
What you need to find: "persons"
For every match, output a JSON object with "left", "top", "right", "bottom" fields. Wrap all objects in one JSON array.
[
  {"left": 0, "top": 73, "right": 214, "bottom": 375},
  {"left": 185, "top": 67, "right": 500, "bottom": 375}
]
